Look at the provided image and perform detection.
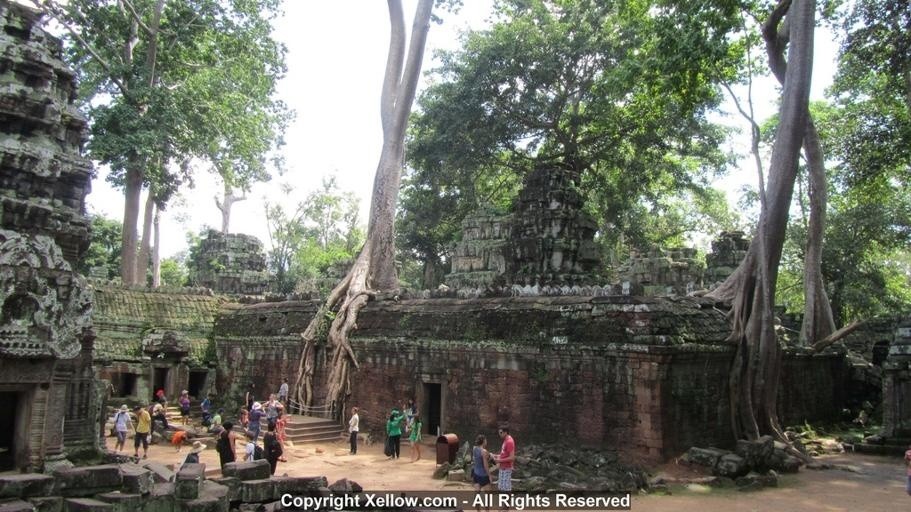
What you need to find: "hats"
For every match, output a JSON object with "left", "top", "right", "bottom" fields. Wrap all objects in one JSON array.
[
  {"left": 217, "top": 409, "right": 224, "bottom": 414},
  {"left": 190, "top": 441, "right": 207, "bottom": 454},
  {"left": 156, "top": 390, "right": 167, "bottom": 402},
  {"left": 118, "top": 405, "right": 129, "bottom": 413},
  {"left": 252, "top": 402, "right": 262, "bottom": 410},
  {"left": 182, "top": 390, "right": 189, "bottom": 395},
  {"left": 244, "top": 431, "right": 255, "bottom": 439},
  {"left": 391, "top": 410, "right": 399, "bottom": 416},
  {"left": 223, "top": 422, "right": 233, "bottom": 429}
]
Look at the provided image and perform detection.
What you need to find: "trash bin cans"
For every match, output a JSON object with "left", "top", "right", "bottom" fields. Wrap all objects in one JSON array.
[{"left": 435, "top": 433, "right": 460, "bottom": 466}]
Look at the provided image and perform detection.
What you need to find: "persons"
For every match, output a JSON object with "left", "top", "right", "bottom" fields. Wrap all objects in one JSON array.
[
  {"left": 471, "top": 433, "right": 496, "bottom": 512},
  {"left": 903, "top": 444, "right": 911, "bottom": 496},
  {"left": 109, "top": 387, "right": 292, "bottom": 477},
  {"left": 489, "top": 424, "right": 517, "bottom": 512},
  {"left": 385, "top": 406, "right": 408, "bottom": 456},
  {"left": 244, "top": 380, "right": 256, "bottom": 411},
  {"left": 278, "top": 376, "right": 290, "bottom": 402},
  {"left": 348, "top": 405, "right": 361, "bottom": 455},
  {"left": 408, "top": 414, "right": 424, "bottom": 462},
  {"left": 402, "top": 396, "right": 422, "bottom": 433}
]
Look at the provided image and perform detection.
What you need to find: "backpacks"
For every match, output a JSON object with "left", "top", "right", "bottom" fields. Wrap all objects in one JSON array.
[
  {"left": 250, "top": 442, "right": 264, "bottom": 460},
  {"left": 147, "top": 405, "right": 154, "bottom": 415}
]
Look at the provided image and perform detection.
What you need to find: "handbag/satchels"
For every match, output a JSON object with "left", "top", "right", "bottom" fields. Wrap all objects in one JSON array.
[
  {"left": 277, "top": 407, "right": 283, "bottom": 418},
  {"left": 384, "top": 435, "right": 394, "bottom": 456},
  {"left": 269, "top": 432, "right": 282, "bottom": 457},
  {"left": 216, "top": 439, "right": 225, "bottom": 452},
  {"left": 112, "top": 426, "right": 117, "bottom": 436}
]
